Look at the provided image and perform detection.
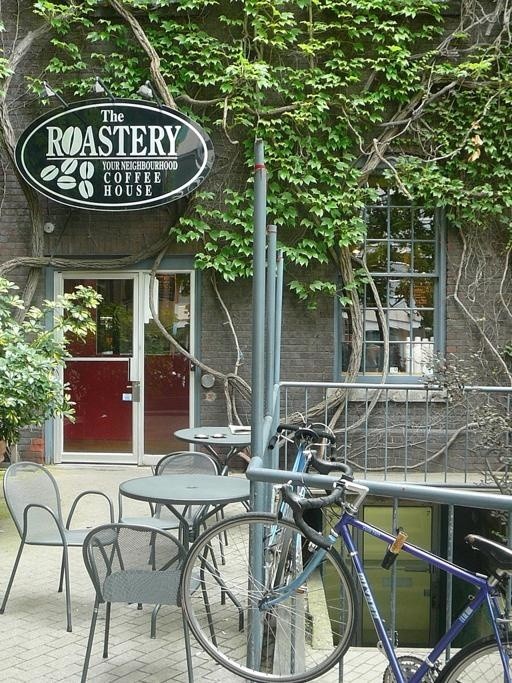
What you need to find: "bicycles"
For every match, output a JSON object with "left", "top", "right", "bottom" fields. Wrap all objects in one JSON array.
[{"left": 181, "top": 424, "right": 512, "bottom": 683}]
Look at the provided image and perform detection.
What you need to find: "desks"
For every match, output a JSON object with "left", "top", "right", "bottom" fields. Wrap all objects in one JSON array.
[
  {"left": 117, "top": 474, "right": 256, "bottom": 640},
  {"left": 173, "top": 426, "right": 252, "bottom": 476}
]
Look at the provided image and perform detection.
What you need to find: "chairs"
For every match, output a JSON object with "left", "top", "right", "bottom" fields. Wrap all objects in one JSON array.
[
  {"left": 148, "top": 450, "right": 229, "bottom": 568},
  {"left": 1, "top": 460, "right": 114, "bottom": 632},
  {"left": 80, "top": 522, "right": 219, "bottom": 682}
]
